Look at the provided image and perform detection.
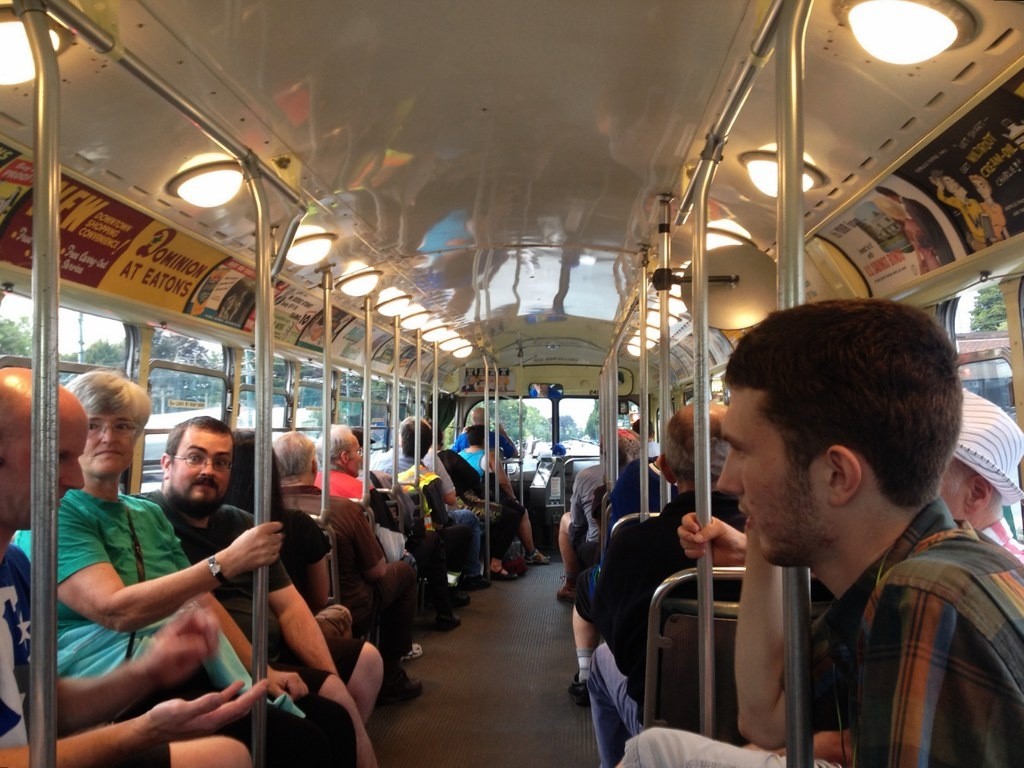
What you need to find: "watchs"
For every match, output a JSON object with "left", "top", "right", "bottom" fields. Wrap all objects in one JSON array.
[{"left": 208, "top": 554, "right": 228, "bottom": 585}]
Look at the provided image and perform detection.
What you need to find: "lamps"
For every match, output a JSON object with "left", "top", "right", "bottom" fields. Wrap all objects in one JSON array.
[
  {"left": 329, "top": 253, "right": 477, "bottom": 362},
  {"left": 736, "top": 142, "right": 833, "bottom": 206},
  {"left": 0, "top": 4, "right": 77, "bottom": 91},
  {"left": 825, "top": 0, "right": 979, "bottom": 72},
  {"left": 282, "top": 219, "right": 339, "bottom": 273},
  {"left": 622, "top": 217, "right": 750, "bottom": 359},
  {"left": 157, "top": 151, "right": 251, "bottom": 210}
]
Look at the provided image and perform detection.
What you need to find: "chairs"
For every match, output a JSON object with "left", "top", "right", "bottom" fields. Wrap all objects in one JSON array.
[{"left": 280, "top": 462, "right": 849, "bottom": 768}]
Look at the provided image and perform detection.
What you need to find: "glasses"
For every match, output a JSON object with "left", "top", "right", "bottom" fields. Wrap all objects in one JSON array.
[
  {"left": 173, "top": 455, "right": 232, "bottom": 472},
  {"left": 345, "top": 448, "right": 362, "bottom": 456},
  {"left": 88, "top": 421, "right": 137, "bottom": 440}
]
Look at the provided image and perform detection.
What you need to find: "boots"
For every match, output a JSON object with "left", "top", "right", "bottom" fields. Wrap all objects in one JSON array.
[{"left": 556, "top": 576, "right": 577, "bottom": 602}]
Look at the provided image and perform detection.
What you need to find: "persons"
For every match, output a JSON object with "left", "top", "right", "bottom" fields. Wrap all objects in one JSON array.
[
  {"left": 716, "top": 300, "right": 1024, "bottom": 767},
  {"left": 939, "top": 384, "right": 1022, "bottom": 562},
  {"left": 223, "top": 428, "right": 352, "bottom": 639},
  {"left": 9, "top": 366, "right": 357, "bottom": 768},
  {"left": 0, "top": 365, "right": 269, "bottom": 768},
  {"left": 125, "top": 413, "right": 383, "bottom": 768},
  {"left": 557, "top": 400, "right": 853, "bottom": 768},
  {"left": 873, "top": 185, "right": 955, "bottom": 276},
  {"left": 272, "top": 404, "right": 551, "bottom": 705}
]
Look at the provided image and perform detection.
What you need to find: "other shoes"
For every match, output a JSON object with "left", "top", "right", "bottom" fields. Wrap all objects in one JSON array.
[{"left": 490, "top": 568, "right": 519, "bottom": 581}]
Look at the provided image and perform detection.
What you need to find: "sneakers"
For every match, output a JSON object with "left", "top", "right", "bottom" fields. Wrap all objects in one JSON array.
[
  {"left": 460, "top": 575, "right": 492, "bottom": 592},
  {"left": 435, "top": 613, "right": 461, "bottom": 632},
  {"left": 376, "top": 680, "right": 422, "bottom": 708},
  {"left": 525, "top": 548, "right": 551, "bottom": 565},
  {"left": 447, "top": 587, "right": 470, "bottom": 607},
  {"left": 569, "top": 670, "right": 590, "bottom": 706},
  {"left": 399, "top": 643, "right": 423, "bottom": 662}
]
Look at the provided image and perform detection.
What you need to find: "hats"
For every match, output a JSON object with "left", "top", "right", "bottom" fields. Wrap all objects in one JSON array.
[
  {"left": 352, "top": 429, "right": 376, "bottom": 447},
  {"left": 950, "top": 387, "right": 1024, "bottom": 507},
  {"left": 550, "top": 443, "right": 566, "bottom": 455}
]
